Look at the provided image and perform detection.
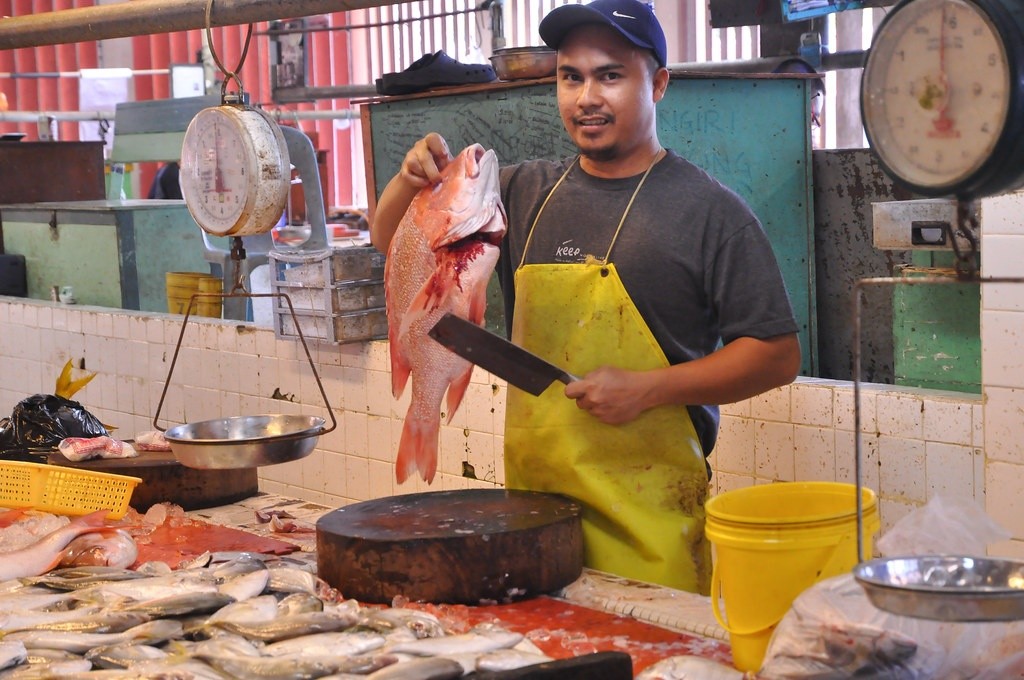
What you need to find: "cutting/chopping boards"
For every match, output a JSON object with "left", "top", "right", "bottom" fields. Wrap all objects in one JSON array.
[
  {"left": 45, "top": 440, "right": 259, "bottom": 516},
  {"left": 315, "top": 488, "right": 585, "bottom": 608}
]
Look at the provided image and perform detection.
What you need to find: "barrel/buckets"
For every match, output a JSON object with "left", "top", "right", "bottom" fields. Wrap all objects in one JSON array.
[
  {"left": 164, "top": 271, "right": 215, "bottom": 315},
  {"left": 702, "top": 481, "right": 882, "bottom": 674},
  {"left": 196, "top": 276, "right": 223, "bottom": 318}
]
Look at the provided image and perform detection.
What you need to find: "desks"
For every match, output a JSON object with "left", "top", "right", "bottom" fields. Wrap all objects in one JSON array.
[{"left": 186, "top": 489, "right": 744, "bottom": 680}]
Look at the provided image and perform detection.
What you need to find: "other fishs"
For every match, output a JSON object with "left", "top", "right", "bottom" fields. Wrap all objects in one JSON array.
[
  {"left": 635, "top": 654, "right": 746, "bottom": 678},
  {"left": 4, "top": 508, "right": 554, "bottom": 680},
  {"left": 383, "top": 141, "right": 508, "bottom": 486}
]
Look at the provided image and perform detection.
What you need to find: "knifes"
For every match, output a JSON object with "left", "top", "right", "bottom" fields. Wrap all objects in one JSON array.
[{"left": 427, "top": 312, "right": 582, "bottom": 396}]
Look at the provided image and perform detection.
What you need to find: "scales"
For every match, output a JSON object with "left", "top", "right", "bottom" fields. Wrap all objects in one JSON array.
[
  {"left": 150, "top": 72, "right": 338, "bottom": 471},
  {"left": 847, "top": 3, "right": 1024, "bottom": 623}
]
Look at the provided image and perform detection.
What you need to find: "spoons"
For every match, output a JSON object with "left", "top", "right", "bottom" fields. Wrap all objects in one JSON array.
[{"left": 464, "top": 648, "right": 634, "bottom": 680}]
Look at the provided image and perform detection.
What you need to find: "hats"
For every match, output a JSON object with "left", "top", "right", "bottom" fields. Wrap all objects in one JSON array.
[{"left": 539, "top": 0, "right": 667, "bottom": 68}]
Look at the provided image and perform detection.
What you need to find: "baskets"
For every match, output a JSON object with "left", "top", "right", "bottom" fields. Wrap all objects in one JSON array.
[{"left": 0, "top": 460, "right": 142, "bottom": 521}]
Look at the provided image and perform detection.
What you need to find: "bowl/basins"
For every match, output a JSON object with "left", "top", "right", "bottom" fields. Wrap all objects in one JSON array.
[
  {"left": 487, "top": 51, "right": 558, "bottom": 81},
  {"left": 492, "top": 45, "right": 555, "bottom": 55}
]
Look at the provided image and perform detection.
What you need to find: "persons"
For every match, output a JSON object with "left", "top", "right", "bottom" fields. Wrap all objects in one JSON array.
[{"left": 368, "top": 0, "right": 804, "bottom": 598}]
